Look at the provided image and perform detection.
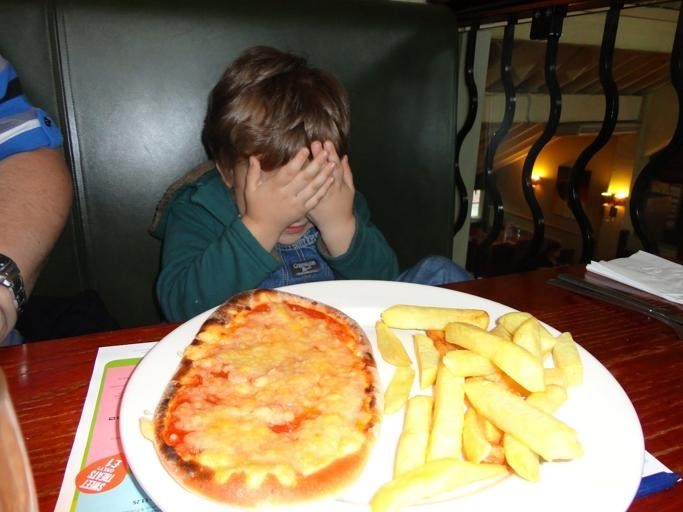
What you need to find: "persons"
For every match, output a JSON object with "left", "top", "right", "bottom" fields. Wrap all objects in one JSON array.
[
  {"left": 0, "top": 49, "right": 75, "bottom": 348},
  {"left": 155, "top": 43, "right": 477, "bottom": 322}
]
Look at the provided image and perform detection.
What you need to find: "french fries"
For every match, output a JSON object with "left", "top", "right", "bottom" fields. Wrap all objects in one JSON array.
[{"left": 359, "top": 304, "right": 588, "bottom": 511}]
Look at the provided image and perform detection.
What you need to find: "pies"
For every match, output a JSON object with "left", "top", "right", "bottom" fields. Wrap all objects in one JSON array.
[{"left": 139, "top": 287, "right": 386, "bottom": 509}]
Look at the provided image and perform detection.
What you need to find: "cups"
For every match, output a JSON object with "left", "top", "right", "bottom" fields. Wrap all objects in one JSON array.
[{"left": 503, "top": 223, "right": 521, "bottom": 241}]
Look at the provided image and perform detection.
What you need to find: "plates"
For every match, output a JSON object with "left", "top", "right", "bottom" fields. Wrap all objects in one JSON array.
[{"left": 118, "top": 277, "right": 644, "bottom": 512}]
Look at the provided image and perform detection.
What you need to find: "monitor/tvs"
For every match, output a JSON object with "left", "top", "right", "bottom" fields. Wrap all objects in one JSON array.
[{"left": 470, "top": 188, "right": 485, "bottom": 223}]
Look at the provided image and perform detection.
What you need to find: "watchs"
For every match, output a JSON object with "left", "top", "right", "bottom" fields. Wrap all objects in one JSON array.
[{"left": 1, "top": 254, "right": 29, "bottom": 315}]
